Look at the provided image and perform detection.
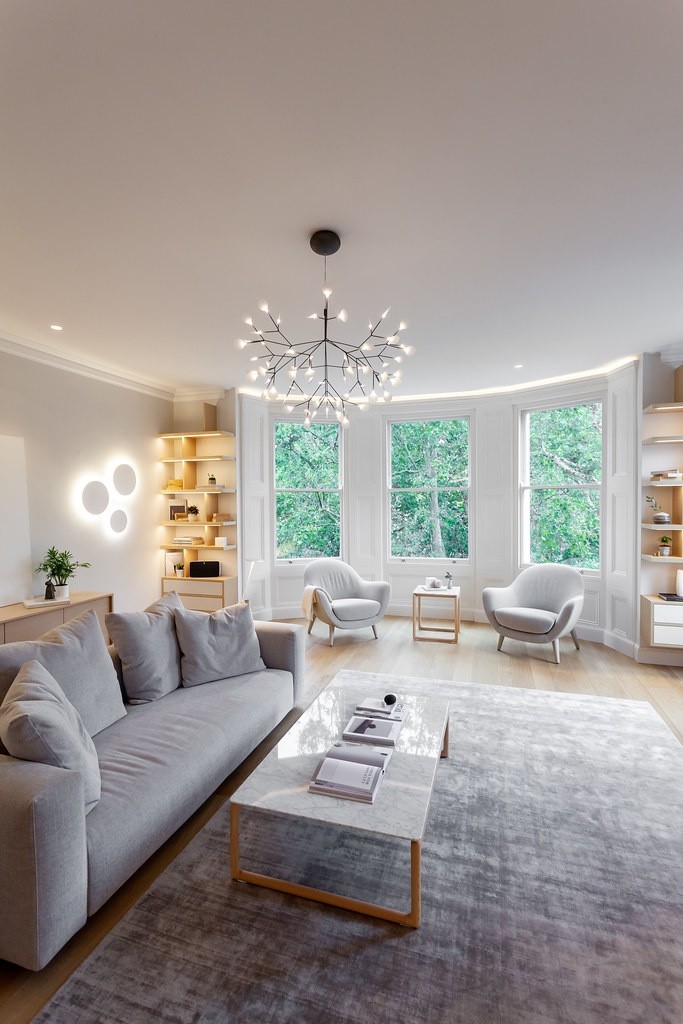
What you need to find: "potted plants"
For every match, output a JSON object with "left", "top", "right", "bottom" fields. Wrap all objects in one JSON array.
[
  {"left": 208, "top": 473, "right": 216, "bottom": 484},
  {"left": 176, "top": 563, "right": 185, "bottom": 577},
  {"left": 645, "top": 494, "right": 671, "bottom": 524},
  {"left": 32, "top": 548, "right": 91, "bottom": 598},
  {"left": 186, "top": 505, "right": 199, "bottom": 521},
  {"left": 658, "top": 536, "right": 672, "bottom": 555}
]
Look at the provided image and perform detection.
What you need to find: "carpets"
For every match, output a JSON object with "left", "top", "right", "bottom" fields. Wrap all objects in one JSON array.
[{"left": 28, "top": 671, "right": 683, "bottom": 1023}]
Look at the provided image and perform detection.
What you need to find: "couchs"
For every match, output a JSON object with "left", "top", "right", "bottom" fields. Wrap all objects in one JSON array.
[{"left": 0, "top": 621, "right": 306, "bottom": 971}]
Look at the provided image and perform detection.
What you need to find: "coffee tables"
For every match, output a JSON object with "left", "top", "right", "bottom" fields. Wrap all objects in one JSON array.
[
  {"left": 230, "top": 684, "right": 449, "bottom": 928},
  {"left": 412, "top": 585, "right": 460, "bottom": 642}
]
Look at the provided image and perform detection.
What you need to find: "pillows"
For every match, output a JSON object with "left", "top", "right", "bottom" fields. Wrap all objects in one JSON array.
[
  {"left": 104, "top": 589, "right": 185, "bottom": 704},
  {"left": 173, "top": 598, "right": 267, "bottom": 687},
  {"left": 0, "top": 608, "right": 129, "bottom": 737},
  {"left": 0, "top": 660, "right": 101, "bottom": 816}
]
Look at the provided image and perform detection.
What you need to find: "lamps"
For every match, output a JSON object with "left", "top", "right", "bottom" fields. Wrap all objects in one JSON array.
[{"left": 238, "top": 229, "right": 416, "bottom": 432}]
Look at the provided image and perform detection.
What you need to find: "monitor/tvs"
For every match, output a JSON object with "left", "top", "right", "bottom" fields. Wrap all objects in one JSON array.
[{"left": 190, "top": 560, "right": 222, "bottom": 578}]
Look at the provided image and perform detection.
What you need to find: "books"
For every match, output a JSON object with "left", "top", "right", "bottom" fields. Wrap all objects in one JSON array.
[
  {"left": 342, "top": 715, "right": 402, "bottom": 746},
  {"left": 173, "top": 537, "right": 204, "bottom": 545},
  {"left": 169, "top": 498, "right": 187, "bottom": 520},
  {"left": 658, "top": 593, "right": 683, "bottom": 602},
  {"left": 650, "top": 469, "right": 683, "bottom": 481},
  {"left": 24, "top": 595, "right": 70, "bottom": 608},
  {"left": 354, "top": 697, "right": 406, "bottom": 721},
  {"left": 308, "top": 740, "right": 393, "bottom": 803}
]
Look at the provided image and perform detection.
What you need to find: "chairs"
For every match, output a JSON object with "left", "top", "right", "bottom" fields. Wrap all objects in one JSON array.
[
  {"left": 483, "top": 562, "right": 584, "bottom": 663},
  {"left": 302, "top": 557, "right": 390, "bottom": 645}
]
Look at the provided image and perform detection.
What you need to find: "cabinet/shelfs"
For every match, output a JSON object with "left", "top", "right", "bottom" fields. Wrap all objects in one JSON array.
[
  {"left": 0, "top": 591, "right": 115, "bottom": 646},
  {"left": 157, "top": 429, "right": 239, "bottom": 613},
  {"left": 641, "top": 400, "right": 683, "bottom": 649}
]
